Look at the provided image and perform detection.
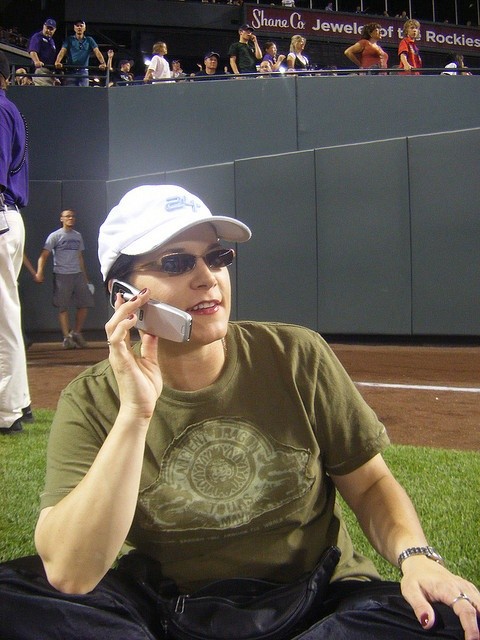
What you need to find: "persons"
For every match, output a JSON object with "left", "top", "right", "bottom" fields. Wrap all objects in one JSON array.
[
  {"left": 0, "top": 47, "right": 32, "bottom": 434},
  {"left": 190, "top": 63, "right": 203, "bottom": 79},
  {"left": 1, "top": 183, "right": 480, "bottom": 640},
  {"left": 438, "top": 55, "right": 463, "bottom": 76},
  {"left": 229, "top": 23, "right": 263, "bottom": 78},
  {"left": 261, "top": 41, "right": 286, "bottom": 77},
  {"left": 28, "top": 19, "right": 57, "bottom": 86},
  {"left": 286, "top": 34, "right": 311, "bottom": 76},
  {"left": 256, "top": 60, "right": 273, "bottom": 79},
  {"left": 171, "top": 60, "right": 187, "bottom": 82},
  {"left": 107, "top": 49, "right": 134, "bottom": 87},
  {"left": 179, "top": 68, "right": 183, "bottom": 73},
  {"left": 128, "top": 72, "right": 134, "bottom": 81},
  {"left": 343, "top": 22, "right": 389, "bottom": 75},
  {"left": 143, "top": 41, "right": 172, "bottom": 84},
  {"left": 15, "top": 68, "right": 32, "bottom": 86},
  {"left": 22, "top": 254, "right": 46, "bottom": 286},
  {"left": 32, "top": 208, "right": 95, "bottom": 350},
  {"left": 54, "top": 18, "right": 107, "bottom": 86},
  {"left": 194, "top": 51, "right": 231, "bottom": 81},
  {"left": 397, "top": 18, "right": 425, "bottom": 75}
]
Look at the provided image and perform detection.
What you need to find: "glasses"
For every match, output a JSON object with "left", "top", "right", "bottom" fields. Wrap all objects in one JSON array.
[
  {"left": 62, "top": 214, "right": 75, "bottom": 217},
  {"left": 122, "top": 248, "right": 236, "bottom": 277}
]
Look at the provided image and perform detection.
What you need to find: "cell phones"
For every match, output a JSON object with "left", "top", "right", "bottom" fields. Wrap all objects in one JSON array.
[{"left": 108, "top": 278, "right": 193, "bottom": 343}]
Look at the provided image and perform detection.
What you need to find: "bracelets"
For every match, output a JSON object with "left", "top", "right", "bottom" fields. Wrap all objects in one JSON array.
[{"left": 100, "top": 61, "right": 106, "bottom": 64}]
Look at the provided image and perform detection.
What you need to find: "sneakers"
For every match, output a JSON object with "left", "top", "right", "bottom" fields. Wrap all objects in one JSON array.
[
  {"left": 21, "top": 406, "right": 33, "bottom": 419},
  {"left": 63, "top": 335, "right": 81, "bottom": 349},
  {"left": 0, "top": 420, "right": 23, "bottom": 433},
  {"left": 71, "top": 331, "right": 88, "bottom": 348}
]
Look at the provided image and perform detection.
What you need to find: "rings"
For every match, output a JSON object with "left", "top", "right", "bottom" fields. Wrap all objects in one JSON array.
[
  {"left": 449, "top": 592, "right": 470, "bottom": 611},
  {"left": 106, "top": 339, "right": 112, "bottom": 345}
]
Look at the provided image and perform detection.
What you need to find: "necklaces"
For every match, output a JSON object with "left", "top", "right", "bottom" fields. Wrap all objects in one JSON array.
[{"left": 221, "top": 337, "right": 229, "bottom": 363}]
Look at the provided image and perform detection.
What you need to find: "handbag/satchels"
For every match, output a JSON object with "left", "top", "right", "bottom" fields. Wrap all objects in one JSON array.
[{"left": 117, "top": 538, "right": 344, "bottom": 638}]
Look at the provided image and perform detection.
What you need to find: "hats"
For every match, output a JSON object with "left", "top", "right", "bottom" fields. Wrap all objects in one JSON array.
[
  {"left": 239, "top": 23, "right": 255, "bottom": 31},
  {"left": 94, "top": 185, "right": 253, "bottom": 282},
  {"left": 73, "top": 16, "right": 86, "bottom": 24},
  {"left": 204, "top": 51, "right": 220, "bottom": 60},
  {"left": 118, "top": 59, "right": 134, "bottom": 68},
  {"left": 45, "top": 18, "right": 56, "bottom": 27}
]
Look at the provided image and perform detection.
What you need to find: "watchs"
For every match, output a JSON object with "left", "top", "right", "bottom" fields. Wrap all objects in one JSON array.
[{"left": 395, "top": 545, "right": 444, "bottom": 571}]
